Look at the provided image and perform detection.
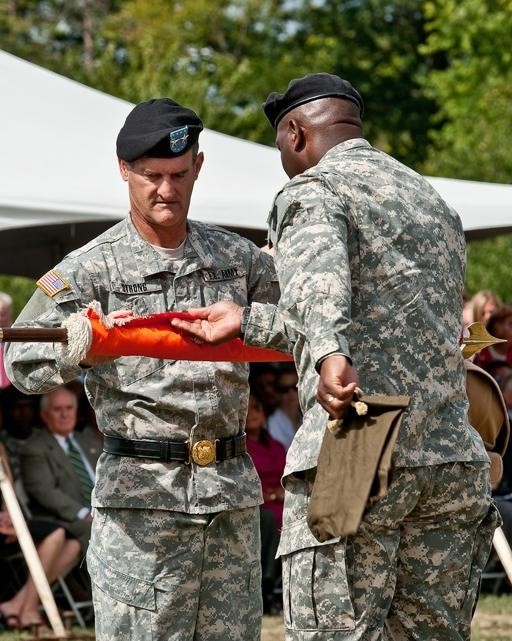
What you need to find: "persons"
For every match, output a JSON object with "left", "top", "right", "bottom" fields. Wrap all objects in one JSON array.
[
  {"left": 3, "top": 97, "right": 281, "bottom": 639},
  {"left": 460, "top": 291, "right": 512, "bottom": 592},
  {"left": 245, "top": 368, "right": 304, "bottom": 614},
  {"left": 2, "top": 387, "right": 104, "bottom": 635},
  {"left": 171, "top": 72, "right": 497, "bottom": 639}
]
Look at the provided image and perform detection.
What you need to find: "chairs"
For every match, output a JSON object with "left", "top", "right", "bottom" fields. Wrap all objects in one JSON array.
[{"left": 10, "top": 446, "right": 97, "bottom": 631}]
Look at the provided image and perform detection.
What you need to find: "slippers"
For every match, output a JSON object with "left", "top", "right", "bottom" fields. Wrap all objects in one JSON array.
[{"left": 1, "top": 613, "right": 56, "bottom": 636}]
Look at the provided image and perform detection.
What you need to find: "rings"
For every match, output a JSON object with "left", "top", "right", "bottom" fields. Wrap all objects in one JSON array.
[{"left": 325, "top": 396, "right": 333, "bottom": 404}]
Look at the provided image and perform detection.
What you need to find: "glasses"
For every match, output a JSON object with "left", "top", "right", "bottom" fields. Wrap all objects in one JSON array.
[{"left": 274, "top": 379, "right": 300, "bottom": 396}]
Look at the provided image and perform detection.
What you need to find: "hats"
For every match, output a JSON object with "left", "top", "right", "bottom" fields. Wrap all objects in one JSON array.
[
  {"left": 116, "top": 98, "right": 204, "bottom": 163},
  {"left": 262, "top": 72, "right": 364, "bottom": 131}
]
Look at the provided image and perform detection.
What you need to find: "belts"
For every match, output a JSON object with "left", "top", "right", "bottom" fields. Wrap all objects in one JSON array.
[{"left": 101, "top": 434, "right": 248, "bottom": 464}]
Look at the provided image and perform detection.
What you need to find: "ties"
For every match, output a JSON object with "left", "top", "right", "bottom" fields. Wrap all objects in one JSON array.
[{"left": 63, "top": 436, "right": 97, "bottom": 513}]
